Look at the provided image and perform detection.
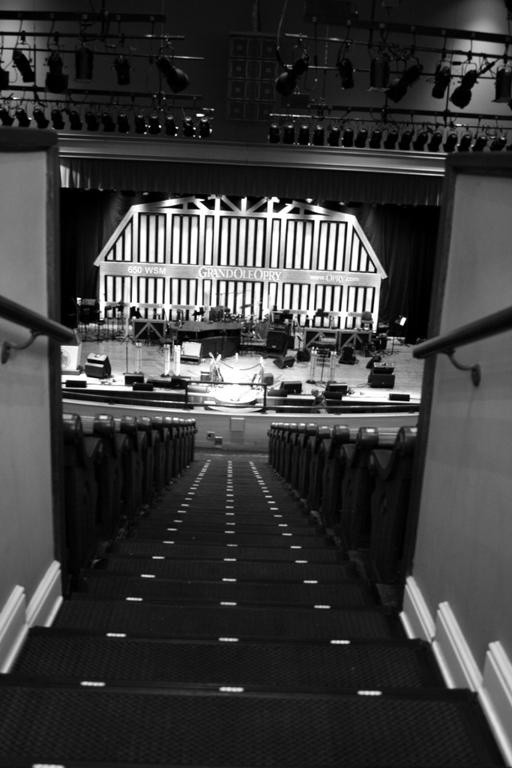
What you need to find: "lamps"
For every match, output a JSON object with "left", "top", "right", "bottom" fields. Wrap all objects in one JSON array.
[
  {"left": 118, "top": 113, "right": 130, "bottom": 133},
  {"left": 368, "top": 56, "right": 390, "bottom": 93},
  {"left": 157, "top": 53, "right": 190, "bottom": 92},
  {"left": 0, "top": 66, "right": 11, "bottom": 90},
  {"left": 135, "top": 112, "right": 147, "bottom": 133},
  {"left": 312, "top": 125, "right": 325, "bottom": 146},
  {"left": 326, "top": 127, "right": 340, "bottom": 146},
  {"left": 383, "top": 129, "right": 399, "bottom": 150},
  {"left": 84, "top": 112, "right": 100, "bottom": 131},
  {"left": 44, "top": 55, "right": 69, "bottom": 94},
  {"left": 427, "top": 133, "right": 442, "bottom": 152},
  {"left": 11, "top": 48, "right": 36, "bottom": 84},
  {"left": 74, "top": 47, "right": 93, "bottom": 84},
  {"left": 102, "top": 112, "right": 116, "bottom": 132},
  {"left": 273, "top": 55, "right": 311, "bottom": 97},
  {"left": 283, "top": 123, "right": 295, "bottom": 144},
  {"left": 493, "top": 64, "right": 512, "bottom": 103},
  {"left": 114, "top": 54, "right": 131, "bottom": 87},
  {"left": 342, "top": 129, "right": 354, "bottom": 147},
  {"left": 443, "top": 130, "right": 511, "bottom": 152},
  {"left": 412, "top": 132, "right": 429, "bottom": 151},
  {"left": 68, "top": 110, "right": 84, "bottom": 130},
  {"left": 15, "top": 108, "right": 31, "bottom": 129},
  {"left": 450, "top": 69, "right": 479, "bottom": 109},
  {"left": 354, "top": 129, "right": 368, "bottom": 148},
  {"left": 51, "top": 109, "right": 65, "bottom": 131},
  {"left": 296, "top": 124, "right": 310, "bottom": 145},
  {"left": 268, "top": 123, "right": 281, "bottom": 144},
  {"left": 32, "top": 108, "right": 50, "bottom": 129},
  {"left": 147, "top": 113, "right": 212, "bottom": 139},
  {"left": 431, "top": 63, "right": 451, "bottom": 100},
  {"left": 397, "top": 131, "right": 412, "bottom": 150},
  {"left": 0, "top": 106, "right": 15, "bottom": 128},
  {"left": 337, "top": 59, "right": 355, "bottom": 89},
  {"left": 369, "top": 129, "right": 382, "bottom": 148},
  {"left": 384, "top": 64, "right": 424, "bottom": 103}
]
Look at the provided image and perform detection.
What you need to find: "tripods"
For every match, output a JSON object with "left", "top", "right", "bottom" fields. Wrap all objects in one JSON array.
[
  {"left": 385, "top": 335, "right": 399, "bottom": 355},
  {"left": 376, "top": 335, "right": 388, "bottom": 357},
  {"left": 120, "top": 307, "right": 135, "bottom": 344}
]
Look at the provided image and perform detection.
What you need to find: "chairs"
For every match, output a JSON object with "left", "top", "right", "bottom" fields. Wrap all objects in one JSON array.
[
  {"left": 265, "top": 419, "right": 418, "bottom": 606},
  {"left": 63, "top": 412, "right": 198, "bottom": 590}
]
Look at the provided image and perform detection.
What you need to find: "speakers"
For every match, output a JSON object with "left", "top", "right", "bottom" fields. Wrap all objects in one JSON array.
[
  {"left": 125, "top": 374, "right": 143, "bottom": 384},
  {"left": 274, "top": 356, "right": 295, "bottom": 368},
  {"left": 367, "top": 374, "right": 395, "bottom": 388},
  {"left": 281, "top": 381, "right": 302, "bottom": 394},
  {"left": 327, "top": 383, "right": 347, "bottom": 396}
]
